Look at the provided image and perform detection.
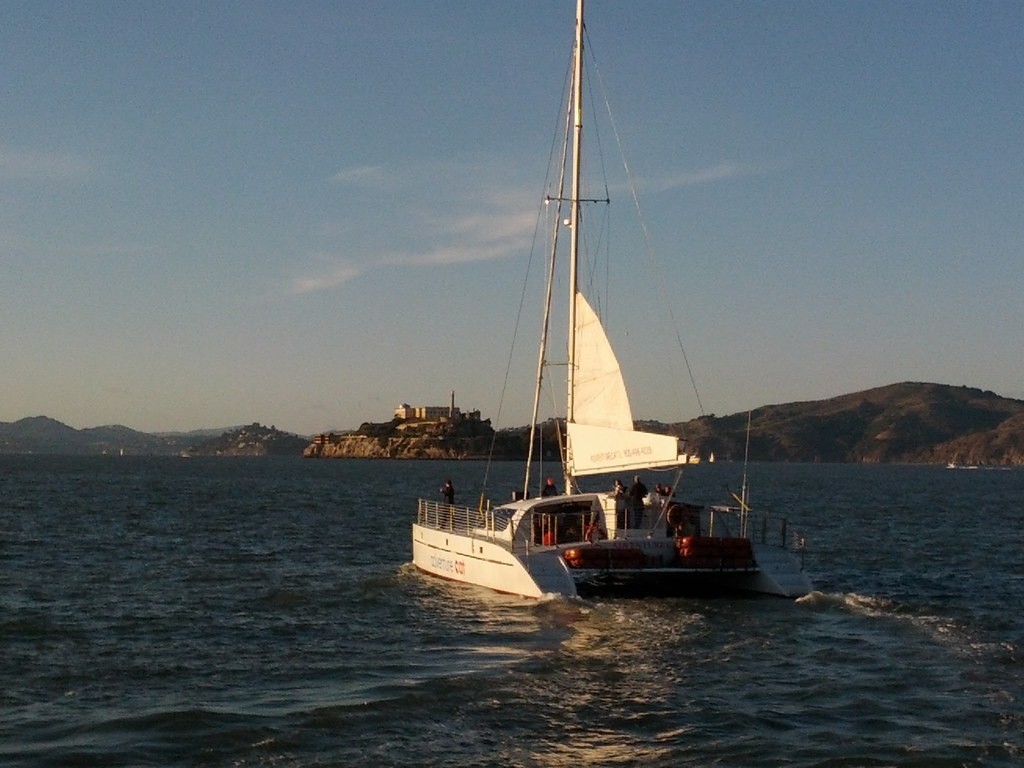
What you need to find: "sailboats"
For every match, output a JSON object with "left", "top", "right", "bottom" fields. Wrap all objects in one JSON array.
[{"left": 407, "top": 1, "right": 814, "bottom": 607}]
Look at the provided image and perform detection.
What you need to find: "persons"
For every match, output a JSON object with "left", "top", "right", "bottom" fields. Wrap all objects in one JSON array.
[
  {"left": 438, "top": 480, "right": 455, "bottom": 530},
  {"left": 542, "top": 477, "right": 558, "bottom": 496},
  {"left": 655, "top": 484, "right": 676, "bottom": 497},
  {"left": 628, "top": 476, "right": 646, "bottom": 528},
  {"left": 616, "top": 479, "right": 625, "bottom": 529}
]
